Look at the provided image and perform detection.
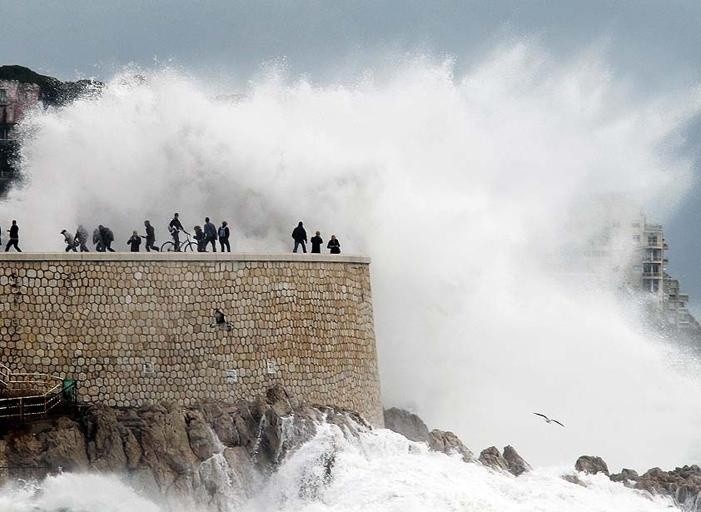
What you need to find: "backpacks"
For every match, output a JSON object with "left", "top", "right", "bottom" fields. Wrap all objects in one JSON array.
[{"left": 220, "top": 226, "right": 227, "bottom": 238}]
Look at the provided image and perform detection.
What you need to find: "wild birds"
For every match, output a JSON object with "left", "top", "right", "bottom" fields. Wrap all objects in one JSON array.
[{"left": 534, "top": 412, "right": 566, "bottom": 427}]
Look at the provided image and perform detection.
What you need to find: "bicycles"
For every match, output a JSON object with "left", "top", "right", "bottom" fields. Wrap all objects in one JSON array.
[{"left": 160, "top": 234, "right": 199, "bottom": 252}]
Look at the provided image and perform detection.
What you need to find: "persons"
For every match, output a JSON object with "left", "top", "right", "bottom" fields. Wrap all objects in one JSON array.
[
  {"left": 203, "top": 216, "right": 218, "bottom": 252},
  {"left": 327, "top": 234, "right": 342, "bottom": 254},
  {"left": 218, "top": 221, "right": 231, "bottom": 252},
  {"left": 292, "top": 221, "right": 308, "bottom": 253},
  {"left": 140, "top": 220, "right": 160, "bottom": 252},
  {"left": 98, "top": 224, "right": 117, "bottom": 252},
  {"left": 5, "top": 219, "right": 22, "bottom": 253},
  {"left": 126, "top": 230, "right": 142, "bottom": 251},
  {"left": 61, "top": 229, "right": 78, "bottom": 252},
  {"left": 93, "top": 228, "right": 106, "bottom": 252},
  {"left": 75, "top": 224, "right": 90, "bottom": 252},
  {"left": 193, "top": 225, "right": 208, "bottom": 251},
  {"left": 168, "top": 212, "right": 190, "bottom": 252},
  {"left": 311, "top": 230, "right": 324, "bottom": 253}
]
